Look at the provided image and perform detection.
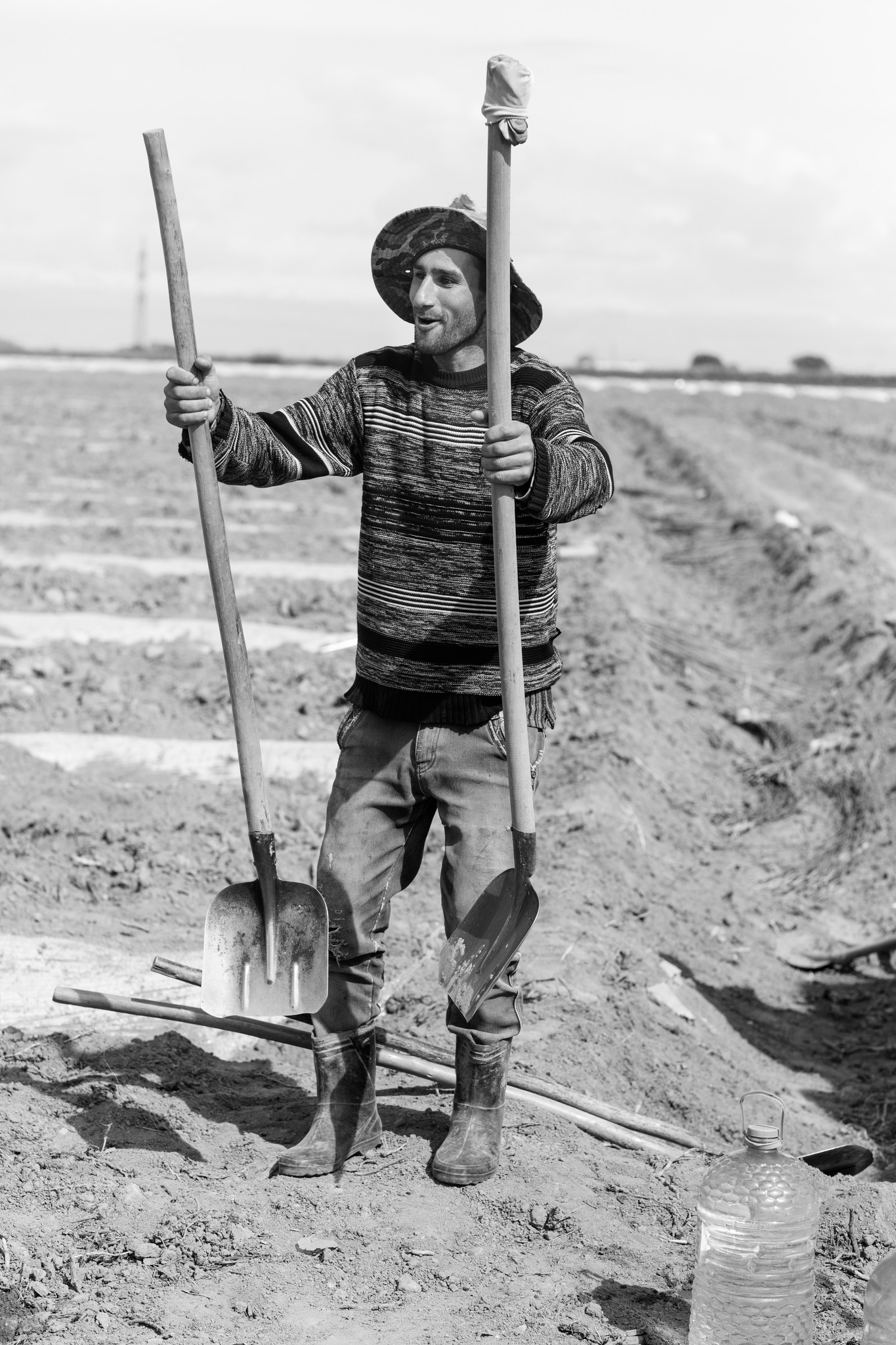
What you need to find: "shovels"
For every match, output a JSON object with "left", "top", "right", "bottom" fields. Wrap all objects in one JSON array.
[
  {"left": 437, "top": 57, "right": 540, "bottom": 1022},
  {"left": 141, "top": 130, "right": 329, "bottom": 1019},
  {"left": 50, "top": 985, "right": 876, "bottom": 1176}
]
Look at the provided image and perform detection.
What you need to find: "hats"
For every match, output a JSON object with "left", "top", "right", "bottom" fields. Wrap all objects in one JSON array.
[{"left": 371, "top": 195, "right": 542, "bottom": 346}]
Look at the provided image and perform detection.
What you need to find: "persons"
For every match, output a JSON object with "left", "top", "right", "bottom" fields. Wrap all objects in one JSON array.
[{"left": 162, "top": 194, "right": 615, "bottom": 1185}]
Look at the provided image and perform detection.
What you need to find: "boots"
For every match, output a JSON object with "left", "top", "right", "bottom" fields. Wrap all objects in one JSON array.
[
  {"left": 431, "top": 1037, "right": 511, "bottom": 1184},
  {"left": 278, "top": 1022, "right": 382, "bottom": 1176}
]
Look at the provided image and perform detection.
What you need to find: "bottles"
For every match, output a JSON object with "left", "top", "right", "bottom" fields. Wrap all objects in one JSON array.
[
  {"left": 862, "top": 1249, "right": 896, "bottom": 1345},
  {"left": 687, "top": 1090, "right": 821, "bottom": 1345}
]
